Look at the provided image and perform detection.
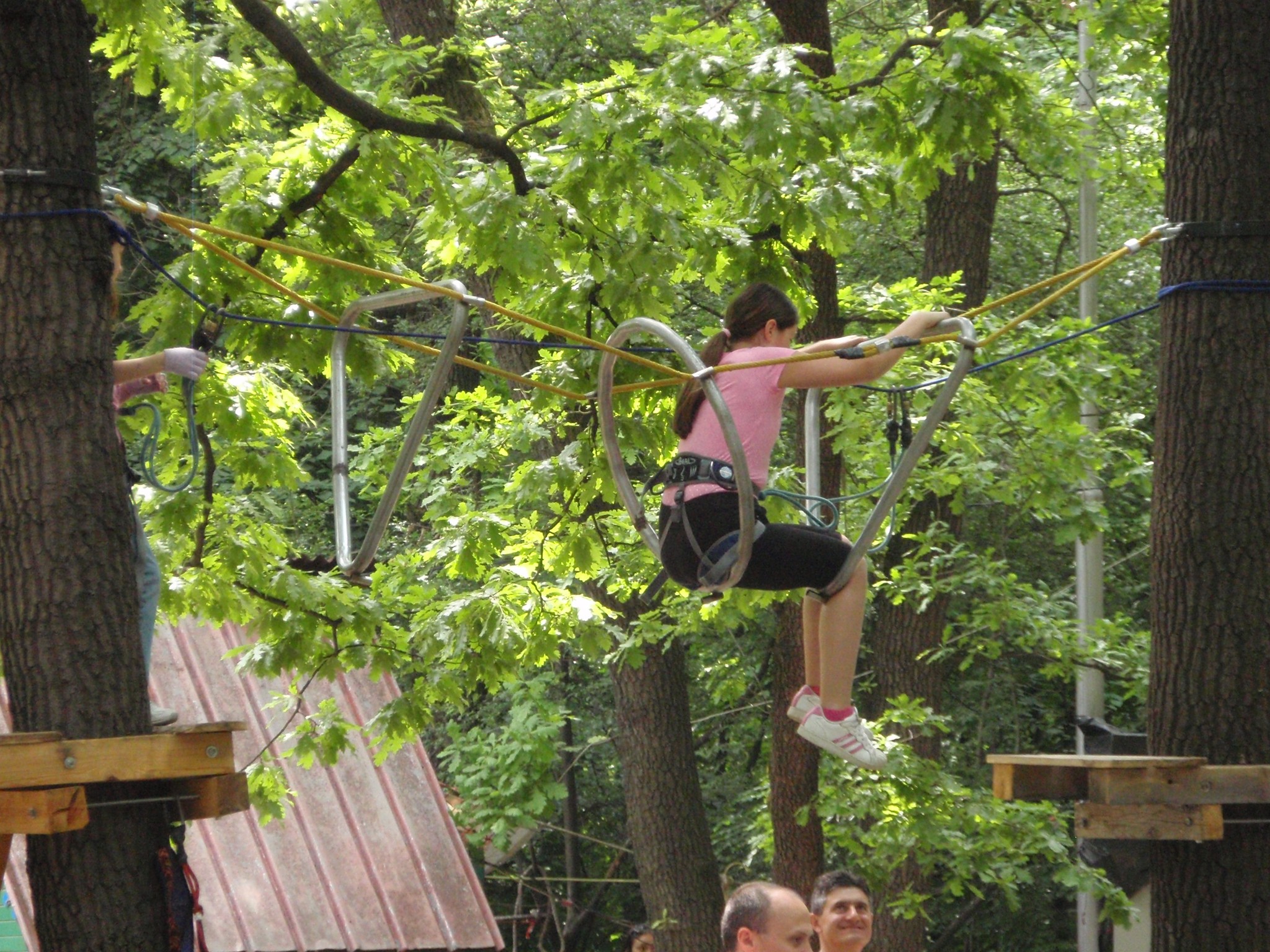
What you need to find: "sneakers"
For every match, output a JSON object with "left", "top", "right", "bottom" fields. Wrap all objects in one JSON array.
[
  {"left": 793, "top": 705, "right": 888, "bottom": 771},
  {"left": 786, "top": 686, "right": 878, "bottom": 745}
]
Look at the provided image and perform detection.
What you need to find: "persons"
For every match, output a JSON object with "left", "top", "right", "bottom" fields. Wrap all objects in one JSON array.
[
  {"left": 811, "top": 870, "right": 874, "bottom": 952},
  {"left": 627, "top": 924, "right": 655, "bottom": 952},
  {"left": 720, "top": 881, "right": 815, "bottom": 952},
  {"left": 658, "top": 281, "right": 948, "bottom": 769},
  {"left": 97, "top": 214, "right": 211, "bottom": 728}
]
